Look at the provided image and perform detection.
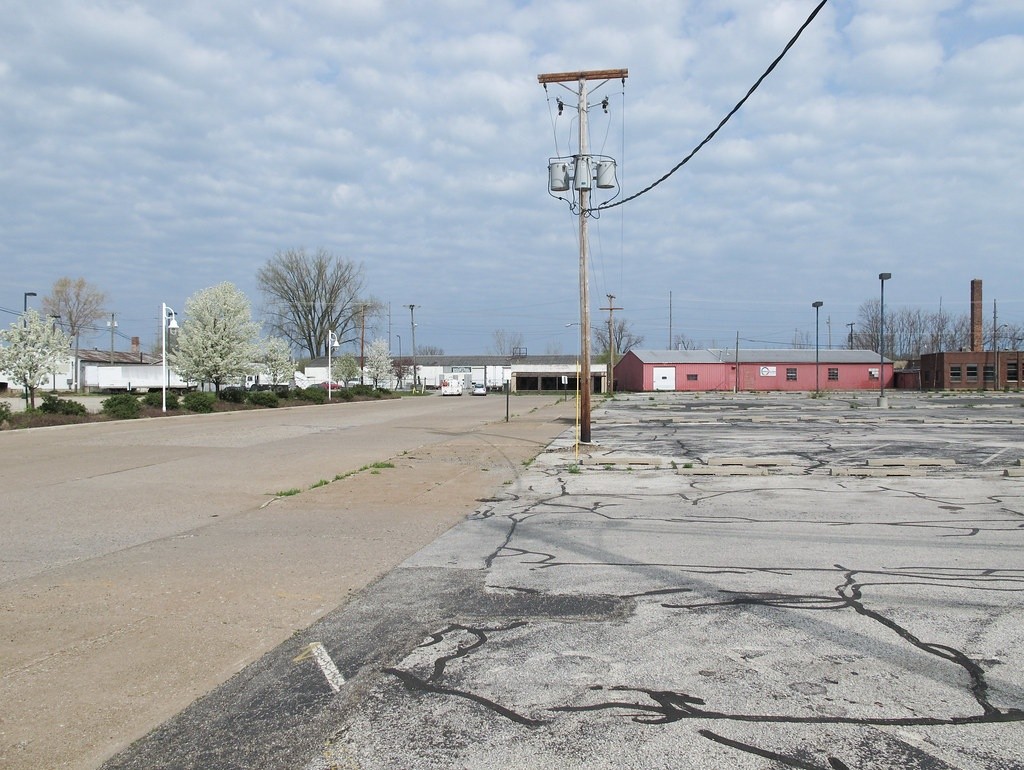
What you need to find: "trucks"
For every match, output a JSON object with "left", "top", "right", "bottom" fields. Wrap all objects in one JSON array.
[
  {"left": 441, "top": 377, "right": 463, "bottom": 397},
  {"left": 348, "top": 377, "right": 374, "bottom": 389},
  {"left": 242, "top": 372, "right": 291, "bottom": 394}
]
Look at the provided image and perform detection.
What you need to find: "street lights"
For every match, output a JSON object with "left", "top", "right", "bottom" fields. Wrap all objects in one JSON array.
[
  {"left": 327, "top": 329, "right": 340, "bottom": 402},
  {"left": 161, "top": 302, "right": 180, "bottom": 412},
  {"left": 878, "top": 273, "right": 893, "bottom": 408},
  {"left": 811, "top": 301, "right": 824, "bottom": 399},
  {"left": 23, "top": 292, "right": 38, "bottom": 413},
  {"left": 50, "top": 314, "right": 62, "bottom": 394},
  {"left": 568, "top": 324, "right": 618, "bottom": 400},
  {"left": 537, "top": 67, "right": 629, "bottom": 455},
  {"left": 411, "top": 324, "right": 420, "bottom": 389},
  {"left": 396, "top": 334, "right": 404, "bottom": 390}
]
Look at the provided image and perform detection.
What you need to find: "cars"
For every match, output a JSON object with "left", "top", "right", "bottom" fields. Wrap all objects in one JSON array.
[
  {"left": 321, "top": 381, "right": 342, "bottom": 390},
  {"left": 473, "top": 384, "right": 487, "bottom": 396}
]
